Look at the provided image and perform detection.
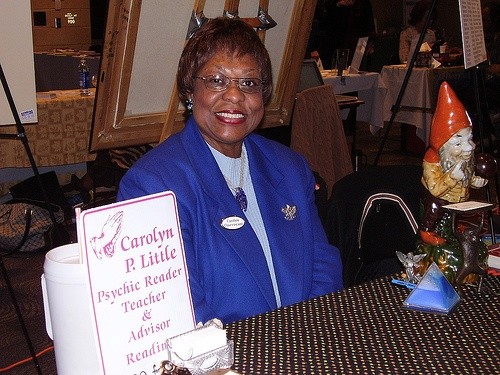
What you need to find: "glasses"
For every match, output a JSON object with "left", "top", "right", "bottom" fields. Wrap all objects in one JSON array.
[{"left": 193, "top": 73, "right": 266, "bottom": 94}]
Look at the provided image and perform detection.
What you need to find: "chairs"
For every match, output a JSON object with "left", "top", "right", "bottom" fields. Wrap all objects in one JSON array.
[{"left": 322, "top": 167, "right": 423, "bottom": 290}]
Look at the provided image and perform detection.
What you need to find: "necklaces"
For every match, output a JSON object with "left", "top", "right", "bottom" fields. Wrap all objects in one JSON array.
[{"left": 213, "top": 144, "right": 248, "bottom": 212}]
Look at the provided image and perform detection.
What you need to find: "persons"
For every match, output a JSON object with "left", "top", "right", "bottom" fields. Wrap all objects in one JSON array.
[
  {"left": 312, "top": 15, "right": 320, "bottom": 63},
  {"left": 114, "top": 16, "right": 345, "bottom": 328},
  {"left": 399, "top": 2, "right": 436, "bottom": 64},
  {"left": 419, "top": 79, "right": 489, "bottom": 247}
]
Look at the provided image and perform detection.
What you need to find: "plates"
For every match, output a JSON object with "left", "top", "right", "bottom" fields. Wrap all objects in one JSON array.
[
  {"left": 320, "top": 69, "right": 349, "bottom": 77},
  {"left": 34, "top": 48, "right": 100, "bottom": 58}
]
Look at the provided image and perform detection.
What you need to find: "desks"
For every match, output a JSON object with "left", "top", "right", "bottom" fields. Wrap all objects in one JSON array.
[
  {"left": 0, "top": 87, "right": 98, "bottom": 169},
  {"left": 221, "top": 267, "right": 500, "bottom": 375},
  {"left": 320, "top": 69, "right": 388, "bottom": 171},
  {"left": 380, "top": 64, "right": 466, "bottom": 155},
  {"left": 35, "top": 53, "right": 101, "bottom": 94}
]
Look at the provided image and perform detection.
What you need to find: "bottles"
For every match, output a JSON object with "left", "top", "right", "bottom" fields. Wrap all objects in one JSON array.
[{"left": 78, "top": 60, "right": 90, "bottom": 94}]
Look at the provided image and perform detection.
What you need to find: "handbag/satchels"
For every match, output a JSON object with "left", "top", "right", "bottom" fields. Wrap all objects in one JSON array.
[{"left": 0, "top": 198, "right": 64, "bottom": 253}]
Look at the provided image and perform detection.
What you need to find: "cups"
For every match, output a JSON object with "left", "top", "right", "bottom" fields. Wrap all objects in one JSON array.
[
  {"left": 92, "top": 75, "right": 98, "bottom": 87},
  {"left": 416, "top": 51, "right": 432, "bottom": 68},
  {"left": 440, "top": 46, "right": 447, "bottom": 53},
  {"left": 439, "top": 52, "right": 449, "bottom": 66},
  {"left": 40, "top": 242, "right": 103, "bottom": 375},
  {"left": 337, "top": 49, "right": 349, "bottom": 70}
]
